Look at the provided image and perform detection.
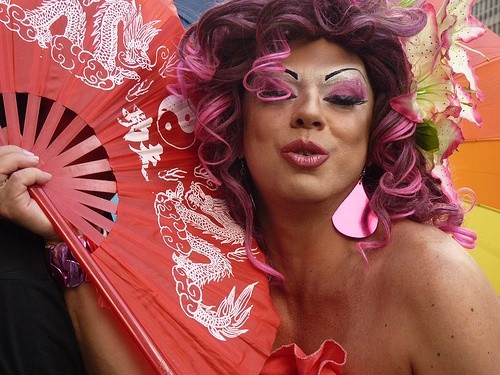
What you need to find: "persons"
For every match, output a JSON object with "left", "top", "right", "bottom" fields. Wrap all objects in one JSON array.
[{"left": 1, "top": 0, "right": 500, "bottom": 375}]
